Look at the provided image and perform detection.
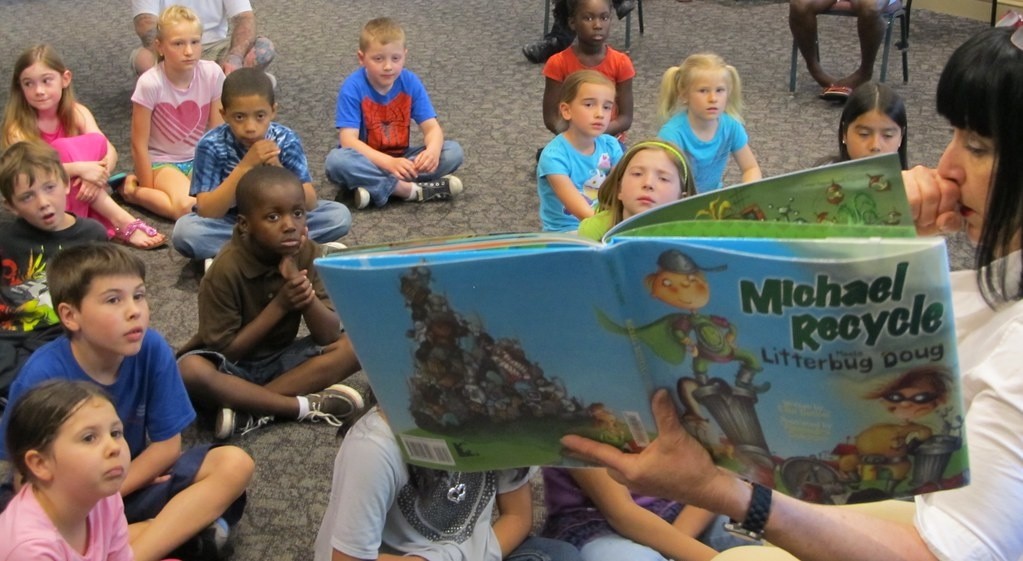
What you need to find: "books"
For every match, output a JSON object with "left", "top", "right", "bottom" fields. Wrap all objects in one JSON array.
[{"left": 312, "top": 150, "right": 971, "bottom": 506}]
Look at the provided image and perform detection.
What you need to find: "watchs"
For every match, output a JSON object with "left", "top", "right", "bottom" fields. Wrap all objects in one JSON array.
[{"left": 723, "top": 478, "right": 772, "bottom": 542}]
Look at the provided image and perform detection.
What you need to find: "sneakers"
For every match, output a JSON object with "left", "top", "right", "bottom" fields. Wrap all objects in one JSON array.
[
  {"left": 412, "top": 172, "right": 463, "bottom": 204},
  {"left": 215, "top": 408, "right": 275, "bottom": 440},
  {"left": 523, "top": 37, "right": 562, "bottom": 64},
  {"left": 612, "top": 0, "right": 638, "bottom": 20},
  {"left": 299, "top": 384, "right": 364, "bottom": 428}
]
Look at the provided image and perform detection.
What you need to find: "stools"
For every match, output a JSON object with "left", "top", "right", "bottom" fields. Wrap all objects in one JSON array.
[{"left": 790, "top": 0, "right": 913, "bottom": 92}]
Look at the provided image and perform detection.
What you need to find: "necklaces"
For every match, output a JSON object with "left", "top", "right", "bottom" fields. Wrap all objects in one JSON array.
[{"left": 446, "top": 471, "right": 467, "bottom": 503}]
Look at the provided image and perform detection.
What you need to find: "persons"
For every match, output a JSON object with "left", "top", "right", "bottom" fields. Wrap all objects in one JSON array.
[
  {"left": 559, "top": 11, "right": 1022, "bottom": 561},
  {"left": 107, "top": 3, "right": 228, "bottom": 220},
  {"left": 577, "top": 136, "right": 697, "bottom": 242},
  {"left": 0, "top": 241, "right": 256, "bottom": 561},
  {"left": 812, "top": 80, "right": 909, "bottom": 170},
  {"left": 0, "top": 44, "right": 170, "bottom": 249},
  {"left": 787, "top": 0, "right": 886, "bottom": 91},
  {"left": 326, "top": 18, "right": 464, "bottom": 210},
  {"left": 535, "top": 70, "right": 625, "bottom": 238},
  {"left": 0, "top": 377, "right": 135, "bottom": 561},
  {"left": 658, "top": 54, "right": 763, "bottom": 193},
  {"left": 129, "top": 0, "right": 277, "bottom": 91},
  {"left": 171, "top": 67, "right": 354, "bottom": 275},
  {"left": 176, "top": 165, "right": 364, "bottom": 441},
  {"left": 313, "top": 403, "right": 764, "bottom": 561},
  {"left": 522, "top": 0, "right": 637, "bottom": 65},
  {"left": 0, "top": 140, "right": 108, "bottom": 413},
  {"left": 542, "top": 0, "right": 637, "bottom": 145}
]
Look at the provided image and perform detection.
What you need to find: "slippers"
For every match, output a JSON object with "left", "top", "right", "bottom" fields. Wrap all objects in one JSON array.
[
  {"left": 110, "top": 217, "right": 167, "bottom": 249},
  {"left": 109, "top": 172, "right": 127, "bottom": 197}
]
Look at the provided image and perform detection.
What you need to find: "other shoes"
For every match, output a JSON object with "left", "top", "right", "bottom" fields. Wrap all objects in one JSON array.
[
  {"left": 819, "top": 84, "right": 853, "bottom": 104},
  {"left": 317, "top": 241, "right": 347, "bottom": 255},
  {"left": 182, "top": 517, "right": 234, "bottom": 558},
  {"left": 204, "top": 257, "right": 216, "bottom": 276},
  {"left": 353, "top": 186, "right": 372, "bottom": 210}
]
